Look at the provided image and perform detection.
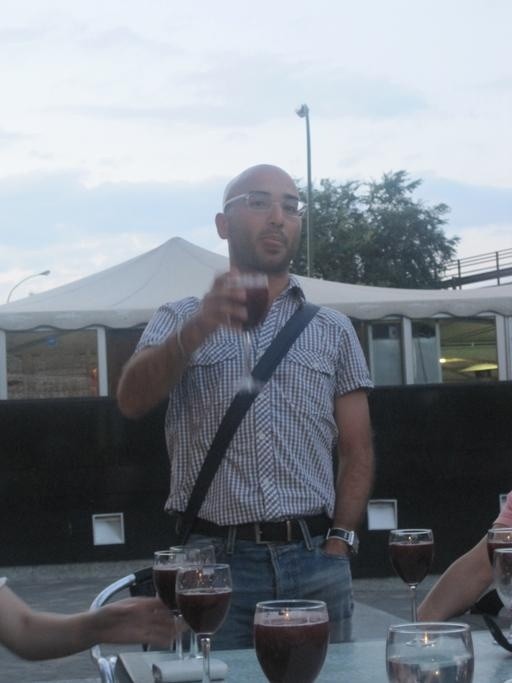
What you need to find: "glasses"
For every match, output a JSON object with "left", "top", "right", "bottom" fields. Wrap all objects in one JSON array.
[{"left": 224, "top": 192, "right": 305, "bottom": 216}]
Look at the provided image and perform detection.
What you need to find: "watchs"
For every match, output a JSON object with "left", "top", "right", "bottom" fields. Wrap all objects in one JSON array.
[{"left": 325, "top": 527, "right": 360, "bottom": 556}]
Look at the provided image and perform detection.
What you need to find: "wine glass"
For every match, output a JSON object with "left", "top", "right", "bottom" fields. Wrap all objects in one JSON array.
[
  {"left": 153, "top": 550, "right": 202, "bottom": 662},
  {"left": 175, "top": 563, "right": 234, "bottom": 682},
  {"left": 492, "top": 548, "right": 512, "bottom": 612},
  {"left": 384, "top": 622, "right": 475, "bottom": 683},
  {"left": 252, "top": 599, "right": 331, "bottom": 682},
  {"left": 388, "top": 528, "right": 434, "bottom": 622},
  {"left": 488, "top": 528, "right": 512, "bottom": 568},
  {"left": 170, "top": 545, "right": 215, "bottom": 660},
  {"left": 224, "top": 272, "right": 270, "bottom": 393}
]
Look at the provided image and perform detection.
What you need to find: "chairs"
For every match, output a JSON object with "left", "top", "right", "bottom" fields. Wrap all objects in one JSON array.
[{"left": 88, "top": 566, "right": 157, "bottom": 682}]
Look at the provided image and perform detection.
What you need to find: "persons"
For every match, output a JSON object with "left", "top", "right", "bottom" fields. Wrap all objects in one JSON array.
[
  {"left": 414, "top": 492, "right": 512, "bottom": 623},
  {"left": 0, "top": 577, "right": 188, "bottom": 661},
  {"left": 118, "top": 163, "right": 378, "bottom": 651}
]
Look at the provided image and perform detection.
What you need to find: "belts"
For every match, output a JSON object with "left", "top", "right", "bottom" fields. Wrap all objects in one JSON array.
[{"left": 192, "top": 515, "right": 334, "bottom": 541}]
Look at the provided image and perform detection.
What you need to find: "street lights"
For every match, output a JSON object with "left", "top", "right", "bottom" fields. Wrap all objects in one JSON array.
[
  {"left": 6, "top": 270, "right": 50, "bottom": 304},
  {"left": 294, "top": 102, "right": 313, "bottom": 279}
]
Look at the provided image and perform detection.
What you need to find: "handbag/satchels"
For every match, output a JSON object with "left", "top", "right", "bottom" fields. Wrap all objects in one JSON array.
[{"left": 130, "top": 567, "right": 157, "bottom": 596}]
